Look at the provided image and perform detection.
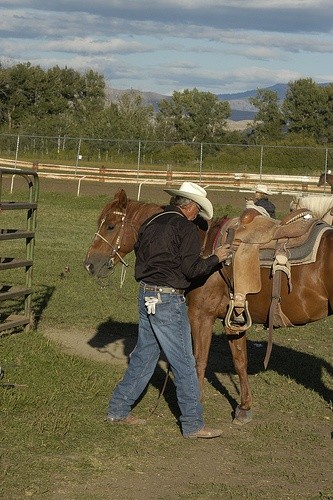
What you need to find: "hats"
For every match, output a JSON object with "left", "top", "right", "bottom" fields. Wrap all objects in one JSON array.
[
  {"left": 163, "top": 182, "right": 213, "bottom": 220},
  {"left": 251, "top": 185, "right": 272, "bottom": 196}
]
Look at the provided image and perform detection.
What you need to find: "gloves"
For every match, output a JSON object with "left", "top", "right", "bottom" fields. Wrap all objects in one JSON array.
[{"left": 144, "top": 297, "right": 158, "bottom": 314}]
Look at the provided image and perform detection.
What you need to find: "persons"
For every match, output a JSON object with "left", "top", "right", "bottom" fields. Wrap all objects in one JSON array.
[
  {"left": 106, "top": 181, "right": 231, "bottom": 438},
  {"left": 255, "top": 184, "right": 276, "bottom": 218}
]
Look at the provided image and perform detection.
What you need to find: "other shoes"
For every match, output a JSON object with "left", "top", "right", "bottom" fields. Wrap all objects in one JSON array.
[
  {"left": 183, "top": 428, "right": 223, "bottom": 438},
  {"left": 107, "top": 414, "right": 146, "bottom": 425}
]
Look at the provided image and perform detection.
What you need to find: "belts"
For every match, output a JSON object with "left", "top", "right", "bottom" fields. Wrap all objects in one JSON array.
[{"left": 140, "top": 284, "right": 183, "bottom": 295}]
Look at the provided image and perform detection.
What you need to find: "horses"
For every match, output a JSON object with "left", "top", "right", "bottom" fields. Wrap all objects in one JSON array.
[{"left": 83, "top": 170, "right": 333, "bottom": 425}]
